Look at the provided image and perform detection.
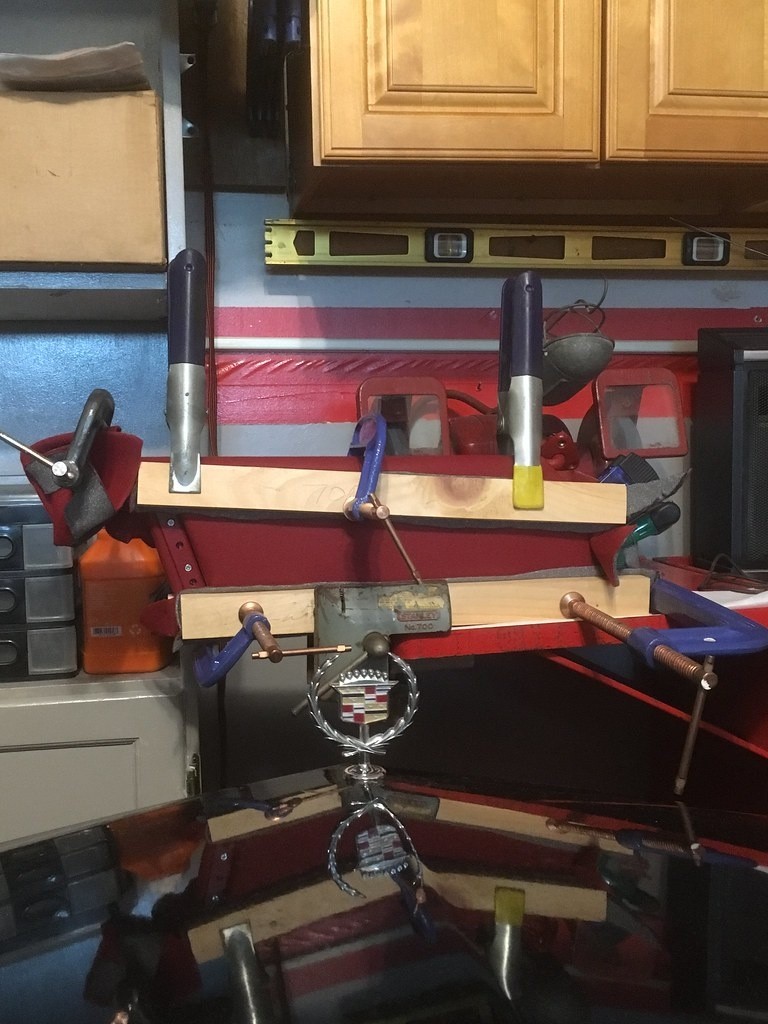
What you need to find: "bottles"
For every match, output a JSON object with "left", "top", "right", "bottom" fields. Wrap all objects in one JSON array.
[
  {"left": 105, "top": 801, "right": 196, "bottom": 892},
  {"left": 80, "top": 523, "right": 165, "bottom": 674}
]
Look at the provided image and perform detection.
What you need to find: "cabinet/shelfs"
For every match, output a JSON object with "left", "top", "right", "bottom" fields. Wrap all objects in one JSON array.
[
  {"left": 284, "top": 0, "right": 768, "bottom": 218},
  {"left": 0, "top": 649, "right": 203, "bottom": 847},
  {"left": 1, "top": 1, "right": 187, "bottom": 322}
]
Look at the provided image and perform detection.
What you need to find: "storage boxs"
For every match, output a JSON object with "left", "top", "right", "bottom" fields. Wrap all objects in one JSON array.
[{"left": 0, "top": 91, "right": 168, "bottom": 271}]
